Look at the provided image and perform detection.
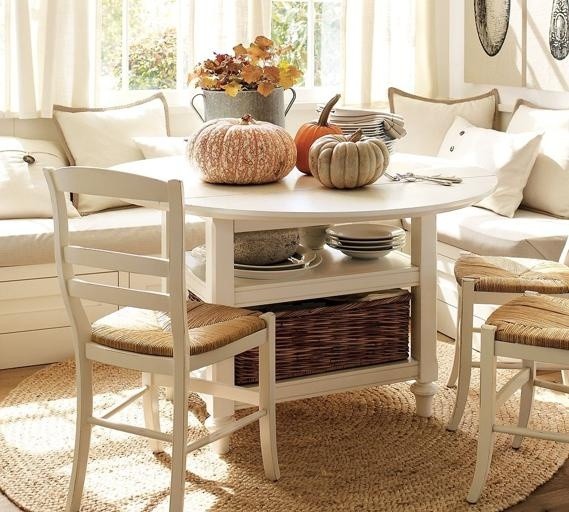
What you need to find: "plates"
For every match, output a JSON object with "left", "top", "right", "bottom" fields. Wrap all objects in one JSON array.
[
  {"left": 324, "top": 223, "right": 407, "bottom": 260},
  {"left": 191, "top": 245, "right": 323, "bottom": 280},
  {"left": 199, "top": 243, "right": 317, "bottom": 268},
  {"left": 309, "top": 118, "right": 399, "bottom": 159}
]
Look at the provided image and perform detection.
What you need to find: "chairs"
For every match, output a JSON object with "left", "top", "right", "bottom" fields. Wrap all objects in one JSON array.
[
  {"left": 446, "top": 237, "right": 569, "bottom": 431},
  {"left": 467, "top": 291, "right": 569, "bottom": 503},
  {"left": 42, "top": 167, "right": 280, "bottom": 512}
]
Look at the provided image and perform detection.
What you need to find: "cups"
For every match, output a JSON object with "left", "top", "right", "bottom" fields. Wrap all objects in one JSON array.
[{"left": 298, "top": 224, "right": 334, "bottom": 250}]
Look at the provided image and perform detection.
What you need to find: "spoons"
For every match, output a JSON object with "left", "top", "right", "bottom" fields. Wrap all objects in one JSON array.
[{"left": 383, "top": 169, "right": 463, "bottom": 183}]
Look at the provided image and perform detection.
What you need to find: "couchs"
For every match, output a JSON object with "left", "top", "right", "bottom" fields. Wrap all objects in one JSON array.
[
  {"left": 0, "top": 114, "right": 321, "bottom": 370},
  {"left": 376, "top": 109, "right": 569, "bottom": 373}
]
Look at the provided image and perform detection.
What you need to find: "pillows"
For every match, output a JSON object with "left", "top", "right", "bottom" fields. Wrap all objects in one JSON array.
[
  {"left": 388, "top": 87, "right": 498, "bottom": 156},
  {"left": 0, "top": 136, "right": 80, "bottom": 218},
  {"left": 130, "top": 136, "right": 189, "bottom": 160},
  {"left": 436, "top": 115, "right": 545, "bottom": 218},
  {"left": 53, "top": 92, "right": 170, "bottom": 216},
  {"left": 506, "top": 99, "right": 569, "bottom": 220}
]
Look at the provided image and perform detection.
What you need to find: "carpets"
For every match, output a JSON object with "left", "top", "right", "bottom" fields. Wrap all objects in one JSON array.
[{"left": 0, "top": 333, "right": 569, "bottom": 512}]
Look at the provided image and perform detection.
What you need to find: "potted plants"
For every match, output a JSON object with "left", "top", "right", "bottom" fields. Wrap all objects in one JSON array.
[{"left": 187, "top": 36, "right": 304, "bottom": 129}]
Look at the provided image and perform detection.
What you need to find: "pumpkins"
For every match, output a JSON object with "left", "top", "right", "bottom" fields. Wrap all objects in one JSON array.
[
  {"left": 293, "top": 93, "right": 343, "bottom": 176},
  {"left": 186, "top": 113, "right": 298, "bottom": 185},
  {"left": 308, "top": 128, "right": 390, "bottom": 188}
]
